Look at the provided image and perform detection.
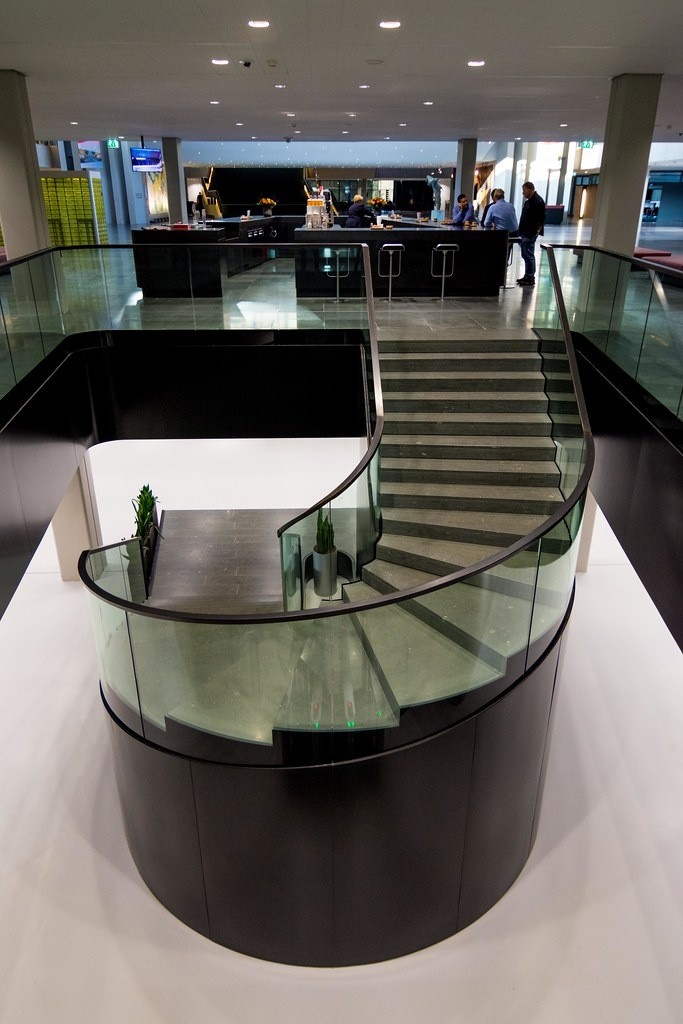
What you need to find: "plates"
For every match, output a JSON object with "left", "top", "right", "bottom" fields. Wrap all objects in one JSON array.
[{"left": 371, "top": 227, "right": 384, "bottom": 230}]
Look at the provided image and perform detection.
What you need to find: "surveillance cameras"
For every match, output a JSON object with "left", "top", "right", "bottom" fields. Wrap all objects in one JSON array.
[
  {"left": 286, "top": 138, "right": 292, "bottom": 142},
  {"left": 244, "top": 62, "right": 251, "bottom": 67},
  {"left": 679, "top": 133, "right": 682, "bottom": 136}
]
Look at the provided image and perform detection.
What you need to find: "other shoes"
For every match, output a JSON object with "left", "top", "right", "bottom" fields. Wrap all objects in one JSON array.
[{"left": 517, "top": 278, "right": 535, "bottom": 286}]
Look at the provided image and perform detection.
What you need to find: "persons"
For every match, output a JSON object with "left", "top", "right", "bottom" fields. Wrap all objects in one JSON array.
[
  {"left": 479, "top": 187, "right": 519, "bottom": 238},
  {"left": 197, "top": 192, "right": 203, "bottom": 203},
  {"left": 346, "top": 194, "right": 375, "bottom": 228},
  {"left": 368, "top": 197, "right": 401, "bottom": 214},
  {"left": 451, "top": 194, "right": 478, "bottom": 224},
  {"left": 517, "top": 181, "right": 547, "bottom": 286}
]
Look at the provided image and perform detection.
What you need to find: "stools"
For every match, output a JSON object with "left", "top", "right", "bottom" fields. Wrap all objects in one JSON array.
[
  {"left": 378, "top": 243, "right": 406, "bottom": 303},
  {"left": 431, "top": 243, "right": 459, "bottom": 303},
  {"left": 502, "top": 236, "right": 523, "bottom": 289},
  {"left": 326, "top": 245, "right": 351, "bottom": 303}
]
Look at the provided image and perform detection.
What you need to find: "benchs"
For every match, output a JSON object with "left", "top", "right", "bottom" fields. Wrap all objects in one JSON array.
[
  {"left": 640, "top": 256, "right": 683, "bottom": 288},
  {"left": 572, "top": 245, "right": 672, "bottom": 270}
]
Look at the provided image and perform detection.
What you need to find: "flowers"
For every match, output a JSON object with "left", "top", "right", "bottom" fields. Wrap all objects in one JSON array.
[
  {"left": 368, "top": 196, "right": 388, "bottom": 209},
  {"left": 256, "top": 196, "right": 277, "bottom": 208}
]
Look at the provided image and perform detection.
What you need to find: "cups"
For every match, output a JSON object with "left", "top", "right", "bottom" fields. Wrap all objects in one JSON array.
[
  {"left": 416, "top": 212, "right": 421, "bottom": 219},
  {"left": 307, "top": 198, "right": 323, "bottom": 206},
  {"left": 308, "top": 223, "right": 312, "bottom": 228}
]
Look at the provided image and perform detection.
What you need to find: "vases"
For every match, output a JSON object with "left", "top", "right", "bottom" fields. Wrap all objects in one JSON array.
[
  {"left": 373, "top": 209, "right": 381, "bottom": 216},
  {"left": 263, "top": 206, "right": 272, "bottom": 217}
]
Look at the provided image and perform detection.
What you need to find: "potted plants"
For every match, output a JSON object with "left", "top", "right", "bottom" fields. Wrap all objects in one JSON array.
[{"left": 313, "top": 506, "right": 338, "bottom": 597}]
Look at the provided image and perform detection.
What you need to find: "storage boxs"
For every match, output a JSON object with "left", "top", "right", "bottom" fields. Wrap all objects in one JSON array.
[{"left": 42, "top": 176, "right": 107, "bottom": 247}]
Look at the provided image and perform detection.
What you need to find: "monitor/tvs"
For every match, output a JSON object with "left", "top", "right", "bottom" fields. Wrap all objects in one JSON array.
[{"left": 130, "top": 148, "right": 163, "bottom": 173}]
